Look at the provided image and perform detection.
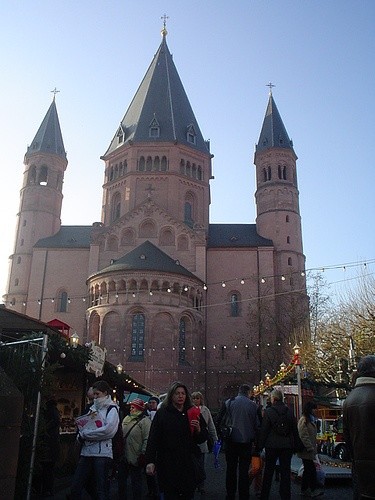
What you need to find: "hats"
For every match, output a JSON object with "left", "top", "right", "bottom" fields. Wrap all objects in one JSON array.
[
  {"left": 149, "top": 397, "right": 159, "bottom": 405},
  {"left": 130, "top": 398, "right": 146, "bottom": 411}
]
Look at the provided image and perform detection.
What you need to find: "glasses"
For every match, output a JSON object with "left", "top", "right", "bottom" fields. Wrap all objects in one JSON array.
[
  {"left": 129, "top": 406, "right": 135, "bottom": 409},
  {"left": 148, "top": 402, "right": 157, "bottom": 404}
]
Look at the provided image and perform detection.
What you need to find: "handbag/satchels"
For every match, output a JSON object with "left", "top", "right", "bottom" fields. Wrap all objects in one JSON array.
[
  {"left": 104, "top": 405, "right": 124, "bottom": 456},
  {"left": 219, "top": 425, "right": 233, "bottom": 442}
]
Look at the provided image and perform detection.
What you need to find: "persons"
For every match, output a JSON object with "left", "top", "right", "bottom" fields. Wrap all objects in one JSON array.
[{"left": 66, "top": 355, "right": 375, "bottom": 500}]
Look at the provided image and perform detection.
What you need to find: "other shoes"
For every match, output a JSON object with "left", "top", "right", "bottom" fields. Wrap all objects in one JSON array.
[
  {"left": 256, "top": 493, "right": 270, "bottom": 500},
  {"left": 66, "top": 494, "right": 73, "bottom": 500},
  {"left": 145, "top": 490, "right": 162, "bottom": 498},
  {"left": 299, "top": 486, "right": 325, "bottom": 498},
  {"left": 279, "top": 494, "right": 291, "bottom": 500}
]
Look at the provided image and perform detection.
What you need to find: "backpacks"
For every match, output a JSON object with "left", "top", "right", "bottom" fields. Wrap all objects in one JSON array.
[{"left": 272, "top": 420, "right": 295, "bottom": 436}]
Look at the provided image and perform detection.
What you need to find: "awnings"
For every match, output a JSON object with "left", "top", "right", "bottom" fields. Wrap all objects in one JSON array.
[{"left": 0, "top": 305, "right": 146, "bottom": 394}]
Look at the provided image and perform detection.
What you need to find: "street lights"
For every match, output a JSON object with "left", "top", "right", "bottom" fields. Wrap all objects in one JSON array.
[
  {"left": 253, "top": 385, "right": 256, "bottom": 394},
  {"left": 259, "top": 380, "right": 264, "bottom": 390},
  {"left": 265, "top": 372, "right": 271, "bottom": 397},
  {"left": 280, "top": 362, "right": 286, "bottom": 403},
  {"left": 292, "top": 343, "right": 303, "bottom": 418}
]
